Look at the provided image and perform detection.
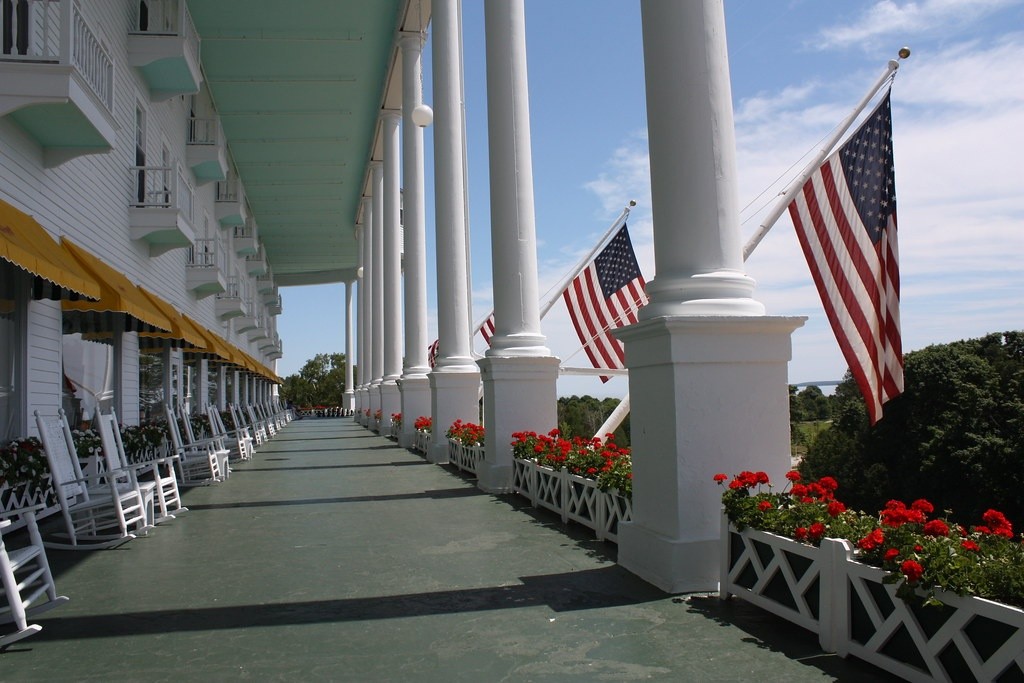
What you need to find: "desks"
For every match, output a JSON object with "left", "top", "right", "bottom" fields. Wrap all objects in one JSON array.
[
  {"left": 88, "top": 481, "right": 157, "bottom": 535},
  {"left": 228, "top": 437, "right": 254, "bottom": 460},
  {"left": 186, "top": 449, "right": 231, "bottom": 482}
]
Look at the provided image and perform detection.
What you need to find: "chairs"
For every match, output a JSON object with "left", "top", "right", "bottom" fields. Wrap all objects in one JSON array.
[
  {"left": 95, "top": 406, "right": 189, "bottom": 526},
  {"left": 205, "top": 400, "right": 294, "bottom": 462},
  {"left": 165, "top": 403, "right": 225, "bottom": 487},
  {"left": 33, "top": 408, "right": 155, "bottom": 550},
  {"left": 0, "top": 503, "right": 70, "bottom": 646},
  {"left": 179, "top": 404, "right": 233, "bottom": 475}
]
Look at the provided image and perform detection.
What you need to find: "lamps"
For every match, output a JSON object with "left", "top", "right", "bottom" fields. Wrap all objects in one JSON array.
[
  {"left": 357, "top": 267, "right": 363, "bottom": 277},
  {"left": 411, "top": 0, "right": 433, "bottom": 127}
]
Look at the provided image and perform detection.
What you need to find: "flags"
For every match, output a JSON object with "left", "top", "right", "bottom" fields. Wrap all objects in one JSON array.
[
  {"left": 428, "top": 339, "right": 439, "bottom": 368},
  {"left": 563, "top": 221, "right": 650, "bottom": 384},
  {"left": 785, "top": 84, "right": 904, "bottom": 427},
  {"left": 480, "top": 313, "right": 495, "bottom": 348}
]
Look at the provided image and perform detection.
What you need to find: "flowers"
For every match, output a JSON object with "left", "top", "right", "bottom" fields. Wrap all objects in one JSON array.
[
  {"left": 357, "top": 407, "right": 431, "bottom": 432},
  {"left": 511, "top": 428, "right": 633, "bottom": 499},
  {"left": 0, "top": 408, "right": 259, "bottom": 493},
  {"left": 713, "top": 463, "right": 1024, "bottom": 608},
  {"left": 446, "top": 418, "right": 485, "bottom": 446}
]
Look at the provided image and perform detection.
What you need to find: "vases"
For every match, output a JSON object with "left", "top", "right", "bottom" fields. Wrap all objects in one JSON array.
[
  {"left": 515, "top": 462, "right": 629, "bottom": 537},
  {"left": 365, "top": 417, "right": 431, "bottom": 452},
  {"left": 730, "top": 531, "right": 1023, "bottom": 683},
  {"left": 449, "top": 441, "right": 487, "bottom": 473},
  {"left": 0, "top": 441, "right": 174, "bottom": 536}
]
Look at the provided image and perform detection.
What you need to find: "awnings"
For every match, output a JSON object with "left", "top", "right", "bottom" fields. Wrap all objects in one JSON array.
[
  {"left": 137, "top": 285, "right": 208, "bottom": 352},
  {"left": 59, "top": 235, "right": 171, "bottom": 334},
  {"left": 234, "top": 347, "right": 284, "bottom": 385},
  {"left": 183, "top": 313, "right": 230, "bottom": 361},
  {"left": 207, "top": 329, "right": 245, "bottom": 368},
  {"left": 0, "top": 198, "right": 104, "bottom": 304}
]
